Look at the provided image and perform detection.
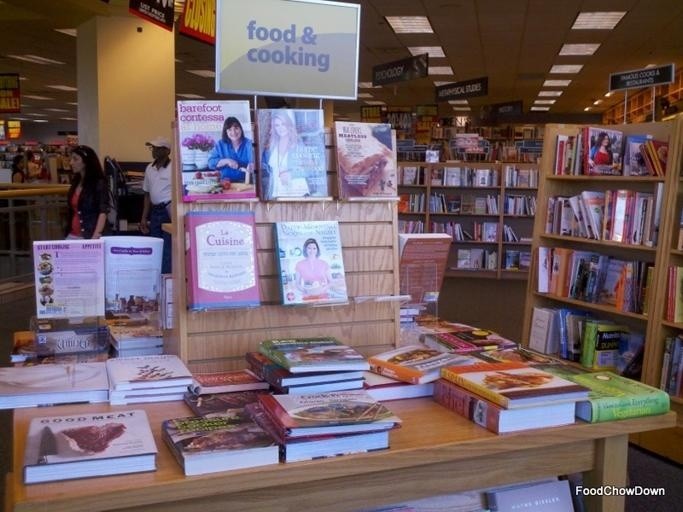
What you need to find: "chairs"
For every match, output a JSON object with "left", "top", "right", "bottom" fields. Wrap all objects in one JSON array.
[{"left": 104, "top": 156, "right": 144, "bottom": 230}]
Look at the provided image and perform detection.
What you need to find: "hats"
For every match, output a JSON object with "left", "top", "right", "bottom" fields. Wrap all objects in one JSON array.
[{"left": 146, "top": 136, "right": 171, "bottom": 153}]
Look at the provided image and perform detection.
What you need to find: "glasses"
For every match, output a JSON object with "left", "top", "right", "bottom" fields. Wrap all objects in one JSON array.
[{"left": 73, "top": 146, "right": 88, "bottom": 157}]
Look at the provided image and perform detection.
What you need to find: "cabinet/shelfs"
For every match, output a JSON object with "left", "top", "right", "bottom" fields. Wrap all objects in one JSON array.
[
  {"left": 397, "top": 125, "right": 542, "bottom": 342},
  {"left": 0, "top": 143, "right": 68, "bottom": 183}
]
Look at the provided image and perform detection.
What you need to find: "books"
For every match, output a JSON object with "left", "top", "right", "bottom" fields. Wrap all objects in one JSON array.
[
  {"left": 184, "top": 210, "right": 262, "bottom": 315},
  {"left": 99, "top": 235, "right": 165, "bottom": 322},
  {"left": 4, "top": 313, "right": 683, "bottom": 512},
  {"left": 176, "top": 99, "right": 259, "bottom": 204},
  {"left": 333, "top": 121, "right": 400, "bottom": 199},
  {"left": 254, "top": 107, "right": 327, "bottom": 201},
  {"left": 30, "top": 239, "right": 105, "bottom": 319},
  {"left": 395, "top": 123, "right": 682, "bottom": 310},
  {"left": 273, "top": 220, "right": 349, "bottom": 306}
]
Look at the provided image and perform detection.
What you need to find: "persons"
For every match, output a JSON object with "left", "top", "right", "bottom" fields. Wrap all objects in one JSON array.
[
  {"left": 292, "top": 236, "right": 336, "bottom": 301},
  {"left": 61, "top": 144, "right": 112, "bottom": 240},
  {"left": 9, "top": 154, "right": 32, "bottom": 251},
  {"left": 207, "top": 115, "right": 255, "bottom": 184},
  {"left": 138, "top": 136, "right": 173, "bottom": 274},
  {"left": 261, "top": 110, "right": 314, "bottom": 199}
]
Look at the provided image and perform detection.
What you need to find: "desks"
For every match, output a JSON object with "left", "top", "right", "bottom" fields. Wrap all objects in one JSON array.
[{"left": 6, "top": 333, "right": 678, "bottom": 512}]
